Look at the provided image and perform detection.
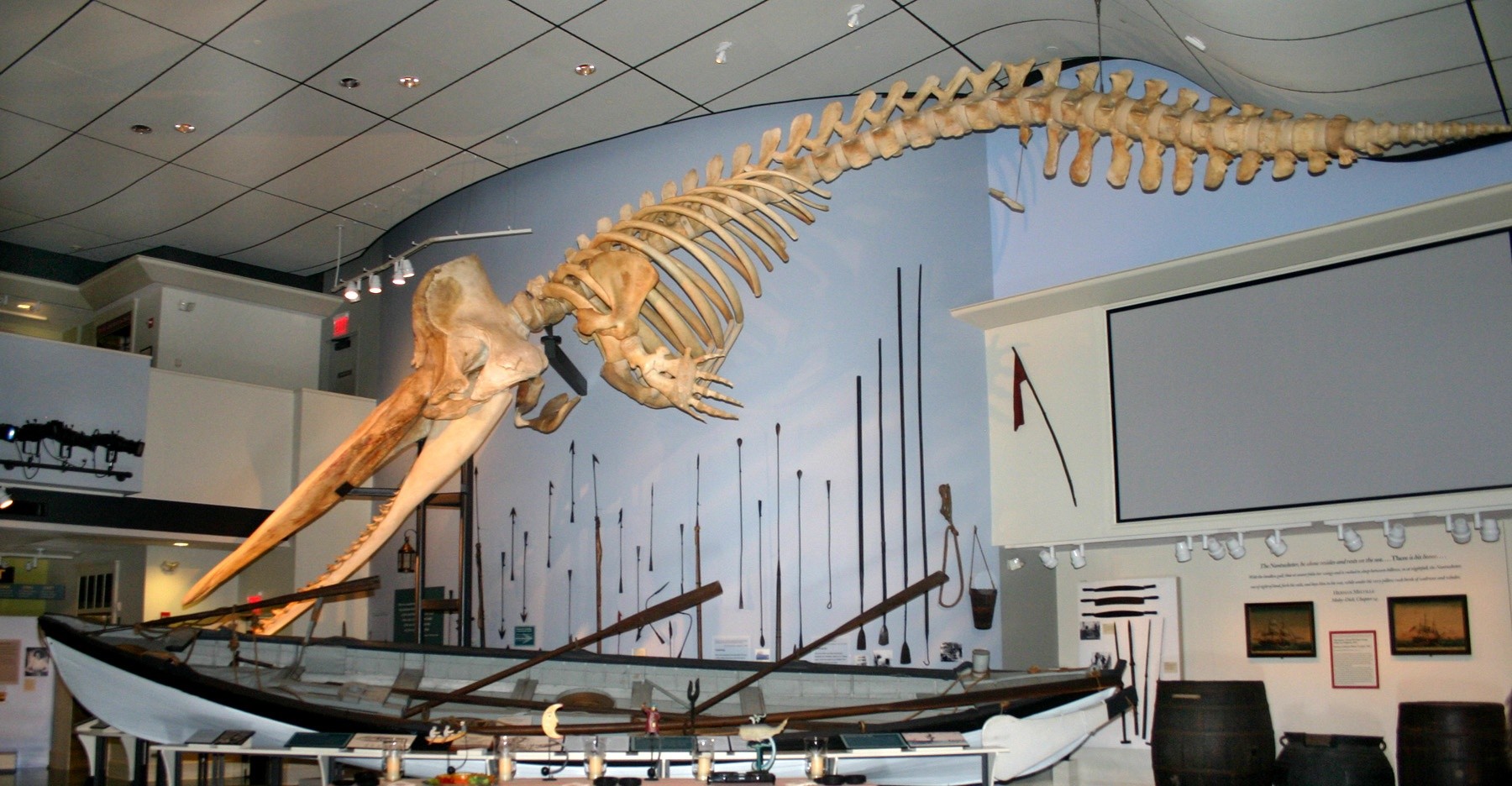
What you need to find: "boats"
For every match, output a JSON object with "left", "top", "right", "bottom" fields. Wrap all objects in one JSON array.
[{"left": 36, "top": 571, "right": 1140, "bottom": 786}]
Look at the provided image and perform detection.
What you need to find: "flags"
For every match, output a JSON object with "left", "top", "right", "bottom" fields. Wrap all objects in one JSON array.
[{"left": 1013, "top": 354, "right": 1027, "bottom": 431}]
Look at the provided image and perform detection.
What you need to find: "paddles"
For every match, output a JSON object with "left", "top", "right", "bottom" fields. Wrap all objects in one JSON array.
[
  {"left": 401, "top": 580, "right": 725, "bottom": 722},
  {"left": 329, "top": 681, "right": 717, "bottom": 719},
  {"left": 94, "top": 574, "right": 382, "bottom": 632},
  {"left": 679, "top": 571, "right": 951, "bottom": 712}
]
